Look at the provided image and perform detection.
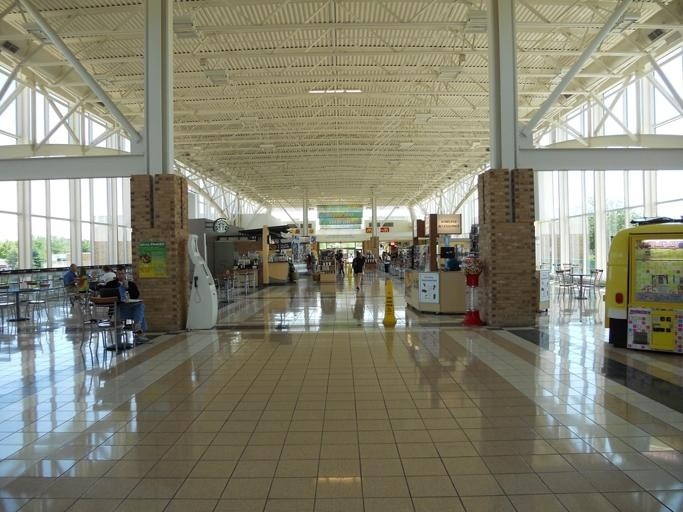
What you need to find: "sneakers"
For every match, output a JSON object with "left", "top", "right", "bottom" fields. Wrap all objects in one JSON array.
[
  {"left": 132, "top": 323, "right": 144, "bottom": 334},
  {"left": 134, "top": 334, "right": 149, "bottom": 342}
]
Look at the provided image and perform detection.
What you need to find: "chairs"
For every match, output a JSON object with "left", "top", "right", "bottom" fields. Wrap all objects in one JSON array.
[
  {"left": 77, "top": 354, "right": 115, "bottom": 403},
  {"left": 556, "top": 299, "right": 603, "bottom": 325},
  {"left": 553, "top": 263, "right": 603, "bottom": 300},
  {"left": 0, "top": 264, "right": 141, "bottom": 353},
  {"left": 213, "top": 269, "right": 258, "bottom": 306}
]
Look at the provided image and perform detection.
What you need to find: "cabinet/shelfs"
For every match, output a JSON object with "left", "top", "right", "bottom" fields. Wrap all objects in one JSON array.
[{"left": 269, "top": 242, "right": 468, "bottom": 314}]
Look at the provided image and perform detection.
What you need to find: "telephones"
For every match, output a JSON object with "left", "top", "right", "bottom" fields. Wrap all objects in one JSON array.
[{"left": 194, "top": 276, "right": 198, "bottom": 287}]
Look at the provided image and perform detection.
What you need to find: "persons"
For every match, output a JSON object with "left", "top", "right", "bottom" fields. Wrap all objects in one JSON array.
[
  {"left": 74, "top": 266, "right": 92, "bottom": 306},
  {"left": 108, "top": 267, "right": 115, "bottom": 273},
  {"left": 351, "top": 252, "right": 366, "bottom": 289},
  {"left": 383, "top": 252, "right": 390, "bottom": 273},
  {"left": 304, "top": 254, "right": 313, "bottom": 275},
  {"left": 325, "top": 247, "right": 344, "bottom": 274},
  {"left": 337, "top": 275, "right": 345, "bottom": 291},
  {"left": 444, "top": 251, "right": 458, "bottom": 268},
  {"left": 63, "top": 263, "right": 81, "bottom": 306},
  {"left": 95, "top": 265, "right": 115, "bottom": 291},
  {"left": 351, "top": 288, "right": 366, "bottom": 326},
  {"left": 101, "top": 265, "right": 152, "bottom": 344}
]
